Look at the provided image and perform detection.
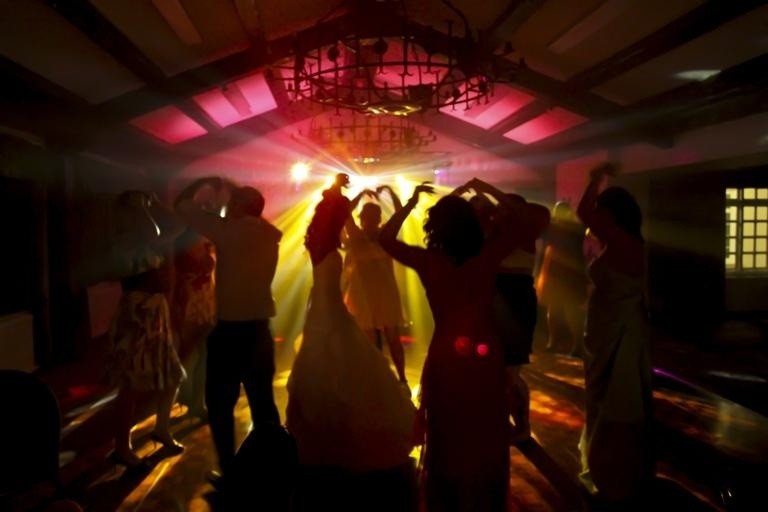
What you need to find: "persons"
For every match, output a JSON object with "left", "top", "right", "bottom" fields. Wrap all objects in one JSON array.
[
  {"left": 379, "top": 175, "right": 549, "bottom": 510},
  {"left": 105, "top": 187, "right": 189, "bottom": 472},
  {"left": 577, "top": 162, "right": 653, "bottom": 503},
  {"left": 451, "top": 177, "right": 549, "bottom": 439},
  {"left": 175, "top": 178, "right": 281, "bottom": 484},
  {"left": 285, "top": 173, "right": 420, "bottom": 478},
  {"left": 341, "top": 184, "right": 412, "bottom": 399},
  {"left": 163, "top": 193, "right": 214, "bottom": 425},
  {"left": 535, "top": 199, "right": 587, "bottom": 349}
]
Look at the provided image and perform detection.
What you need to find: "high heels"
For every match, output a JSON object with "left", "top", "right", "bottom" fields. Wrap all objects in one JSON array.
[
  {"left": 189, "top": 407, "right": 209, "bottom": 424},
  {"left": 110, "top": 451, "right": 152, "bottom": 473},
  {"left": 151, "top": 430, "right": 184, "bottom": 452},
  {"left": 176, "top": 391, "right": 206, "bottom": 415}
]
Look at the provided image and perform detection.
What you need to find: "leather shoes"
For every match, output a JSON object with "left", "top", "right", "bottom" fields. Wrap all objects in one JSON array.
[
  {"left": 544, "top": 343, "right": 560, "bottom": 353},
  {"left": 206, "top": 470, "right": 222, "bottom": 485},
  {"left": 510, "top": 425, "right": 530, "bottom": 441},
  {"left": 572, "top": 350, "right": 592, "bottom": 359}
]
